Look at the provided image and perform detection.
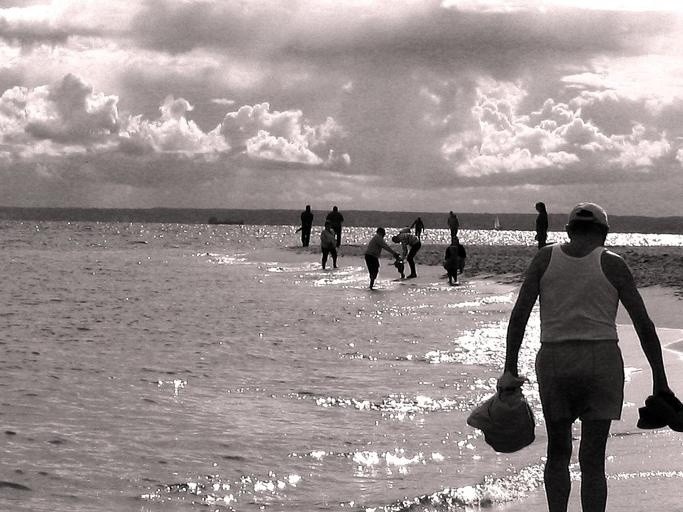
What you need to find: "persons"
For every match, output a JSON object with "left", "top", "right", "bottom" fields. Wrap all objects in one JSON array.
[
  {"left": 301, "top": 205, "right": 314, "bottom": 247},
  {"left": 535, "top": 202, "right": 548, "bottom": 250},
  {"left": 393, "top": 217, "right": 424, "bottom": 278},
  {"left": 444, "top": 211, "right": 466, "bottom": 283},
  {"left": 365, "top": 227, "right": 400, "bottom": 290},
  {"left": 320, "top": 206, "right": 344, "bottom": 269},
  {"left": 504, "top": 202, "right": 674, "bottom": 512}
]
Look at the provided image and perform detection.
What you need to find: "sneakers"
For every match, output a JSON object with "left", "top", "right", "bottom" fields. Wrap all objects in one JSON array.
[{"left": 637, "top": 393, "right": 683, "bottom": 432}]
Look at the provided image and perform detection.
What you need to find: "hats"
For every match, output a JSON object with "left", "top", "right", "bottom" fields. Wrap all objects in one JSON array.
[{"left": 568, "top": 202, "right": 610, "bottom": 228}]
